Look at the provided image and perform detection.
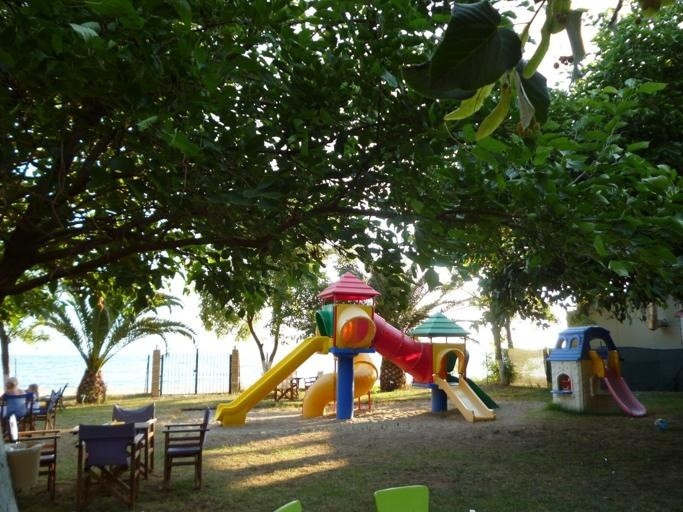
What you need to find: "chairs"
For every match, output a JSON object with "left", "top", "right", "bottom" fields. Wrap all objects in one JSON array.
[
  {"left": 163, "top": 408, "right": 212, "bottom": 489},
  {"left": 1, "top": 377, "right": 68, "bottom": 435},
  {"left": 70, "top": 402, "right": 158, "bottom": 509},
  {"left": 371, "top": 484, "right": 434, "bottom": 509},
  {"left": 7, "top": 413, "right": 63, "bottom": 502}
]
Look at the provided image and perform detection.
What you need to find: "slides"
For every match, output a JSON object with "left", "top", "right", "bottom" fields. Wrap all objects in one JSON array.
[
  {"left": 302, "top": 353, "right": 378, "bottom": 418},
  {"left": 604, "top": 369, "right": 647, "bottom": 417},
  {"left": 432, "top": 373, "right": 496, "bottom": 423},
  {"left": 214, "top": 336, "right": 330, "bottom": 427},
  {"left": 449, "top": 376, "right": 500, "bottom": 409}
]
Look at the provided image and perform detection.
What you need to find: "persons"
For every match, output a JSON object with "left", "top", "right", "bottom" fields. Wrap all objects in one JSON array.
[
  {"left": 27, "top": 384, "right": 40, "bottom": 414},
  {"left": 0, "top": 376, "right": 26, "bottom": 417}
]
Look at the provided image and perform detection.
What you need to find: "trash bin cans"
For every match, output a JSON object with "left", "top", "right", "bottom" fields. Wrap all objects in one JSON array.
[{"left": 4, "top": 440, "right": 46, "bottom": 493}]
